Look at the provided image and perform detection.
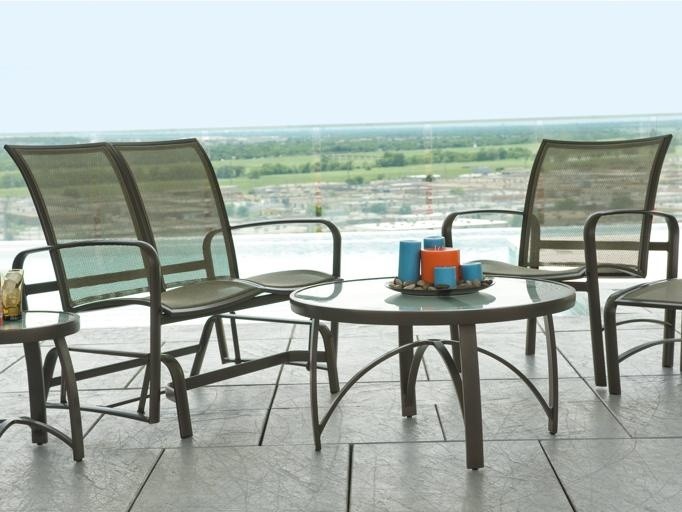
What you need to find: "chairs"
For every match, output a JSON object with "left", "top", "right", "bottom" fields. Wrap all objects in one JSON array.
[
  {"left": 5, "top": 142, "right": 342, "bottom": 440},
  {"left": 604, "top": 272, "right": 681, "bottom": 397},
  {"left": 440, "top": 131, "right": 675, "bottom": 386}
]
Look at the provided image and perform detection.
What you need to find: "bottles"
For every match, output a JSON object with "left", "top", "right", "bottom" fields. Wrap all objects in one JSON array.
[{"left": 0, "top": 269, "right": 23, "bottom": 321}]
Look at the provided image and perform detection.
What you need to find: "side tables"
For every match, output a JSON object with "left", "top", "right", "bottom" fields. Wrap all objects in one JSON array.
[{"left": 0, "top": 311, "right": 85, "bottom": 461}]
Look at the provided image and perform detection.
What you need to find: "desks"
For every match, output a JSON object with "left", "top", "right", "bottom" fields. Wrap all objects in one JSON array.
[{"left": 289, "top": 278, "right": 576, "bottom": 468}]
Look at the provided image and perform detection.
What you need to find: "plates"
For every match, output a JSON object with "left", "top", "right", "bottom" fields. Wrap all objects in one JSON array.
[{"left": 383, "top": 278, "right": 497, "bottom": 297}]
